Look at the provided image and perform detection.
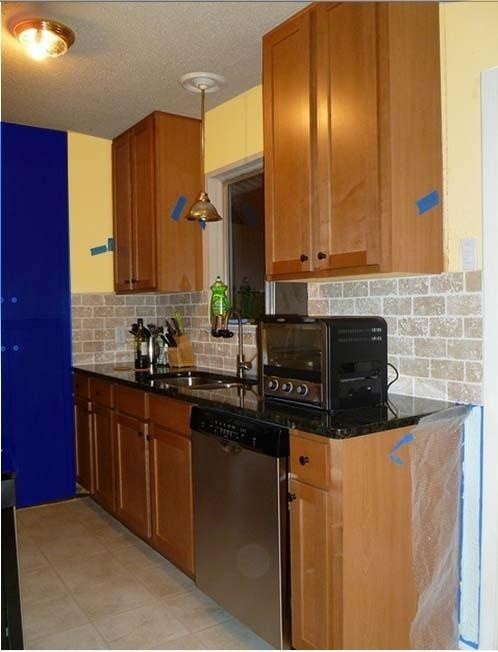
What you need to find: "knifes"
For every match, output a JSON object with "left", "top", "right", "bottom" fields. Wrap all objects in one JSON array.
[{"left": 162, "top": 318, "right": 182, "bottom": 348}]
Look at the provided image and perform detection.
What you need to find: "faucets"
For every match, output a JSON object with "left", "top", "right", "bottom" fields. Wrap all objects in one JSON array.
[{"left": 218, "top": 309, "right": 252, "bottom": 378}]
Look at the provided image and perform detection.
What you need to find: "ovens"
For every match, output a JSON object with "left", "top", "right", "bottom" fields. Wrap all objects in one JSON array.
[{"left": 189, "top": 405, "right": 292, "bottom": 635}]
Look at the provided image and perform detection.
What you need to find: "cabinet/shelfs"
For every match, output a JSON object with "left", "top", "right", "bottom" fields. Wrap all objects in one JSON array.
[
  {"left": 116, "top": 382, "right": 197, "bottom": 579},
  {"left": 0, "top": 121, "right": 75, "bottom": 510},
  {"left": 262, "top": 0, "right": 444, "bottom": 283},
  {"left": 72, "top": 369, "right": 116, "bottom": 517},
  {"left": 112, "top": 110, "right": 203, "bottom": 295},
  {"left": 288, "top": 413, "right": 461, "bottom": 650}
]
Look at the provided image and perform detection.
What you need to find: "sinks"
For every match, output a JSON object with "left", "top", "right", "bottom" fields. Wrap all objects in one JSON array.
[{"left": 146, "top": 371, "right": 257, "bottom": 390}]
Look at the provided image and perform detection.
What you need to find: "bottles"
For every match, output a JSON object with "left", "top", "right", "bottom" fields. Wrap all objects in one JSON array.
[{"left": 133, "top": 318, "right": 164, "bottom": 369}]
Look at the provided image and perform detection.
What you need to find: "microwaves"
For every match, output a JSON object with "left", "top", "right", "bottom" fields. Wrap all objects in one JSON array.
[{"left": 256, "top": 313, "right": 388, "bottom": 411}]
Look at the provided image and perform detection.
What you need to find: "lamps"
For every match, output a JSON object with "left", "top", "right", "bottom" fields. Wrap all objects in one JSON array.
[{"left": 12, "top": 18, "right": 75, "bottom": 61}]
